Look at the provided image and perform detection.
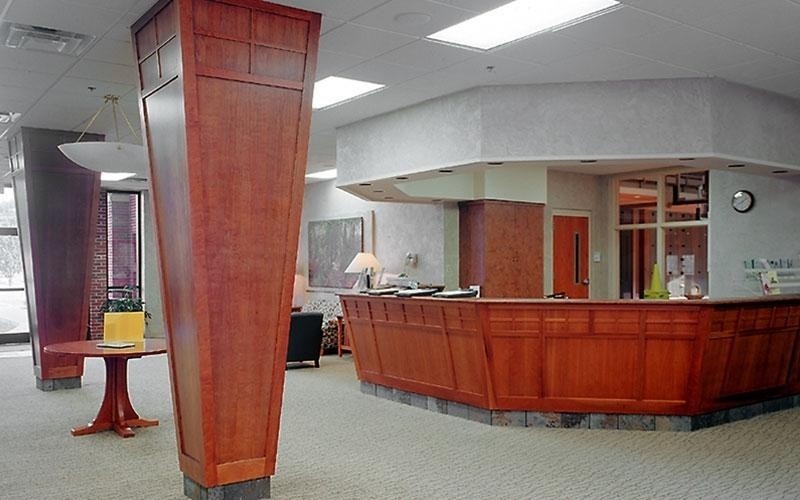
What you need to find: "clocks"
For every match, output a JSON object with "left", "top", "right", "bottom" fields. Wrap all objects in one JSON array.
[{"left": 731, "top": 190, "right": 755, "bottom": 213}]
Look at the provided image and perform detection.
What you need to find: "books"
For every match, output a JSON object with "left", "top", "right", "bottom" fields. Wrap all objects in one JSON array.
[
  {"left": 96, "top": 340, "right": 135, "bottom": 349},
  {"left": 757, "top": 270, "right": 783, "bottom": 297}
]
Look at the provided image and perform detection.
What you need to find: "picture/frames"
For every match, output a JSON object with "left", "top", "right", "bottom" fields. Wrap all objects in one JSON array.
[{"left": 304, "top": 209, "right": 373, "bottom": 292}]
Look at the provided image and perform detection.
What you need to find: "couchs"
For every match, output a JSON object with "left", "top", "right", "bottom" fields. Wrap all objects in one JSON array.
[{"left": 300, "top": 300, "right": 344, "bottom": 356}]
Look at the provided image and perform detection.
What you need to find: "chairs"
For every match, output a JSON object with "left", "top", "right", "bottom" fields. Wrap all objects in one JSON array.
[{"left": 286, "top": 313, "right": 323, "bottom": 370}]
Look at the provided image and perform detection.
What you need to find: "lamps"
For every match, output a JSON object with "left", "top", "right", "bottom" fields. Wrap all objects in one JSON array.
[
  {"left": 57, "top": 95, "right": 146, "bottom": 173},
  {"left": 398, "top": 252, "right": 417, "bottom": 277},
  {"left": 344, "top": 252, "right": 382, "bottom": 292}
]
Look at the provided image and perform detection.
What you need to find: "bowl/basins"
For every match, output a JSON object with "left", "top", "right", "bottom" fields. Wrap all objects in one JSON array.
[{"left": 685, "top": 294, "right": 705, "bottom": 300}]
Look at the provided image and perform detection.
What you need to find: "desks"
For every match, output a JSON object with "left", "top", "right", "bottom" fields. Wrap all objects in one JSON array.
[{"left": 43, "top": 338, "right": 168, "bottom": 438}]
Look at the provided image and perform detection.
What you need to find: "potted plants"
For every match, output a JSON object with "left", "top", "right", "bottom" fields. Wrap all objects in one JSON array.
[{"left": 97, "top": 287, "right": 151, "bottom": 341}]
